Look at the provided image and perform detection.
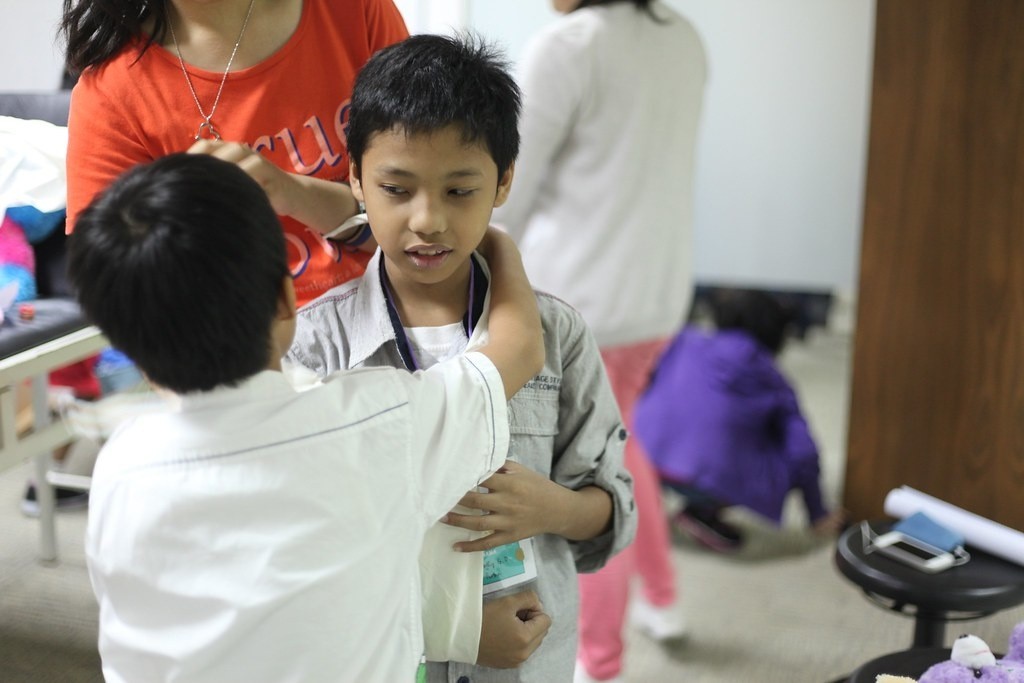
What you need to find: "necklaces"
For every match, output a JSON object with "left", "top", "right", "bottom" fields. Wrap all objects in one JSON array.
[{"left": 167, "top": 0, "right": 255, "bottom": 141}]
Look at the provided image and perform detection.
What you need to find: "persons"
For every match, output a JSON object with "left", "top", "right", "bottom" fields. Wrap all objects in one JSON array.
[{"left": 65, "top": 0, "right": 833, "bottom": 683}]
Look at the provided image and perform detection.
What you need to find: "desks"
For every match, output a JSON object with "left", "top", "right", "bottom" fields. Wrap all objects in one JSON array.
[{"left": 0, "top": 300, "right": 108, "bottom": 563}]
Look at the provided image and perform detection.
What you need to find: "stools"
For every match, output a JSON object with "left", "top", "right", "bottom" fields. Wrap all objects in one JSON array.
[{"left": 833, "top": 518, "right": 1023, "bottom": 681}]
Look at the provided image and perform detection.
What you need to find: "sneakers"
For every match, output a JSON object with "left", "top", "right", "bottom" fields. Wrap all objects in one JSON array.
[
  {"left": 572, "top": 662, "right": 617, "bottom": 683},
  {"left": 676, "top": 509, "right": 745, "bottom": 552},
  {"left": 627, "top": 601, "right": 687, "bottom": 642}
]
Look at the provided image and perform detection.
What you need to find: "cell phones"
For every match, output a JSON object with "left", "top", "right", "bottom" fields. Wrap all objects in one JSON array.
[{"left": 874, "top": 530, "right": 953, "bottom": 573}]
